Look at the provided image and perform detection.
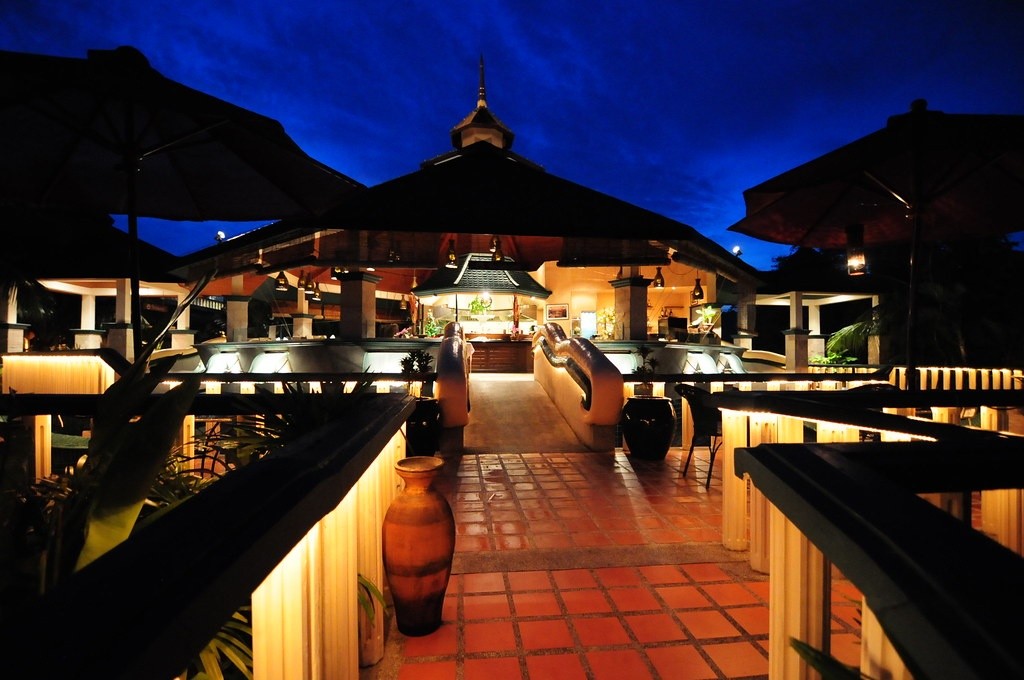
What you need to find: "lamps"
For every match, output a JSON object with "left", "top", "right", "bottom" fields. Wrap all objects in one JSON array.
[
  {"left": 654, "top": 266, "right": 664, "bottom": 289},
  {"left": 692, "top": 270, "right": 704, "bottom": 301},
  {"left": 444, "top": 233, "right": 459, "bottom": 268},
  {"left": 492, "top": 236, "right": 504, "bottom": 261},
  {"left": 844, "top": 222, "right": 867, "bottom": 276},
  {"left": 275, "top": 270, "right": 288, "bottom": 291},
  {"left": 400, "top": 295, "right": 408, "bottom": 310},
  {"left": 411, "top": 269, "right": 418, "bottom": 288},
  {"left": 298, "top": 266, "right": 349, "bottom": 301}
]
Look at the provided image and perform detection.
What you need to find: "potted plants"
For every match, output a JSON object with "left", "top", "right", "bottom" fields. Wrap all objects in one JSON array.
[
  {"left": 621, "top": 344, "right": 676, "bottom": 462},
  {"left": 401, "top": 348, "right": 443, "bottom": 458},
  {"left": 701, "top": 306, "right": 716, "bottom": 331}
]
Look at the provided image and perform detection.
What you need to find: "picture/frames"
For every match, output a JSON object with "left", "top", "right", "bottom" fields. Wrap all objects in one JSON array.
[{"left": 547, "top": 304, "right": 569, "bottom": 320}]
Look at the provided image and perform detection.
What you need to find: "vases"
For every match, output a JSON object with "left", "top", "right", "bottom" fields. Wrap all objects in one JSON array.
[{"left": 383, "top": 456, "right": 455, "bottom": 636}]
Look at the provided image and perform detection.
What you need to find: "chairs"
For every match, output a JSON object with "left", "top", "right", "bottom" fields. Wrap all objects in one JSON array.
[{"left": 674, "top": 383, "right": 723, "bottom": 488}]
